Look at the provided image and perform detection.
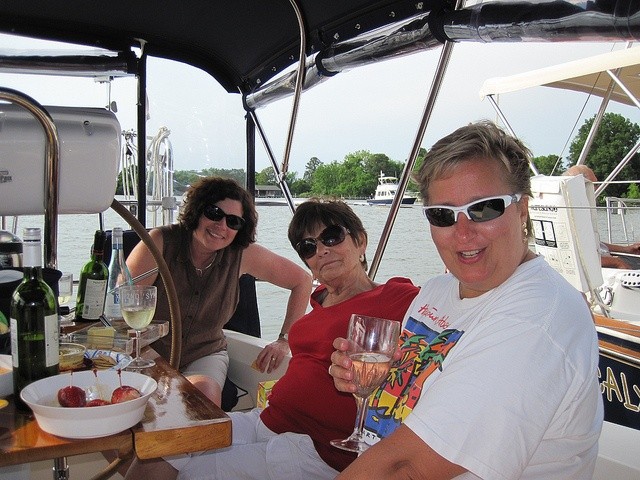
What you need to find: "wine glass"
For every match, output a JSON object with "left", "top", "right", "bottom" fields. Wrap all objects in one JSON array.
[
  {"left": 330, "top": 314, "right": 400, "bottom": 453},
  {"left": 119, "top": 285, "right": 157, "bottom": 368}
]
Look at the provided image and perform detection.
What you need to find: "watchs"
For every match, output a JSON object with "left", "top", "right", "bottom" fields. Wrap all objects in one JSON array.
[{"left": 279, "top": 333, "right": 288, "bottom": 341}]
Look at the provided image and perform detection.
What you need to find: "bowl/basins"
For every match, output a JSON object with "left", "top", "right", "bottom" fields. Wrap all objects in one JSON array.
[
  {"left": 59, "top": 343, "right": 87, "bottom": 371},
  {"left": 19, "top": 370, "right": 158, "bottom": 439}
]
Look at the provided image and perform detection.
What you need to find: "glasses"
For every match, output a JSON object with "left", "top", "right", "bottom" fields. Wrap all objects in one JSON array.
[
  {"left": 203, "top": 203, "right": 247, "bottom": 230},
  {"left": 296, "top": 224, "right": 352, "bottom": 260},
  {"left": 422, "top": 193, "right": 523, "bottom": 227}
]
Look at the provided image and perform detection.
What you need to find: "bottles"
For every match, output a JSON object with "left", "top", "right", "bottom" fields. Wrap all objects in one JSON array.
[
  {"left": 10, "top": 227, "right": 59, "bottom": 413},
  {"left": 103, "top": 228, "right": 134, "bottom": 321},
  {"left": 75, "top": 230, "right": 109, "bottom": 322}
]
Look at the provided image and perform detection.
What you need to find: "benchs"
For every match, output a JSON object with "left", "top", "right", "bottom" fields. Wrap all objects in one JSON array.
[{"left": 221, "top": 329, "right": 640, "bottom": 479}]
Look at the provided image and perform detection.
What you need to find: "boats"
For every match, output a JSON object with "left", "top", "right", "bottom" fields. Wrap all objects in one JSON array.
[
  {"left": 476, "top": 40, "right": 640, "bottom": 474},
  {"left": 366, "top": 170, "right": 417, "bottom": 208}
]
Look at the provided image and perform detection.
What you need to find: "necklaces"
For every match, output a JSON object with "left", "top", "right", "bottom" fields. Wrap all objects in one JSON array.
[{"left": 195, "top": 251, "right": 217, "bottom": 278}]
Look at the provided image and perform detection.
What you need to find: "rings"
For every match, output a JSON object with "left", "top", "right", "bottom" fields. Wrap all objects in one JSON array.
[
  {"left": 328, "top": 364, "right": 336, "bottom": 376},
  {"left": 271, "top": 358, "right": 276, "bottom": 361}
]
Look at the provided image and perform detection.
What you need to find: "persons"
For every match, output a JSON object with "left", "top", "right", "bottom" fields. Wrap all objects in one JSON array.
[
  {"left": 159, "top": 195, "right": 420, "bottom": 480},
  {"left": 328, "top": 120, "right": 606, "bottom": 480},
  {"left": 114, "top": 176, "right": 313, "bottom": 408},
  {"left": 560, "top": 164, "right": 640, "bottom": 270}
]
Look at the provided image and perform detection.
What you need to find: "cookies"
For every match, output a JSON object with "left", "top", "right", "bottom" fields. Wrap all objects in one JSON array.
[{"left": 92, "top": 354, "right": 115, "bottom": 370}]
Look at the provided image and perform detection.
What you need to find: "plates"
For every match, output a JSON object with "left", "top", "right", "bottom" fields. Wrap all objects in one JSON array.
[{"left": 84, "top": 349, "right": 133, "bottom": 371}]
